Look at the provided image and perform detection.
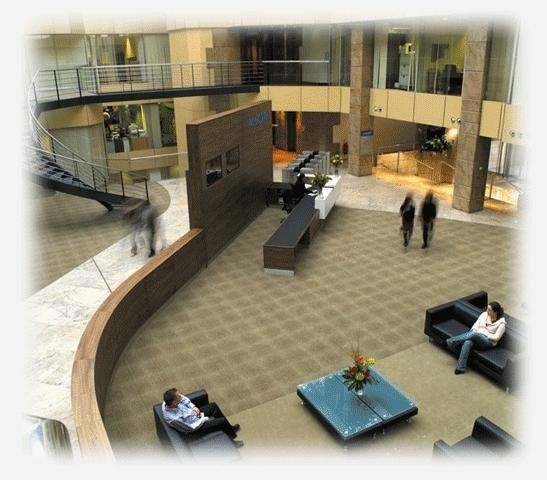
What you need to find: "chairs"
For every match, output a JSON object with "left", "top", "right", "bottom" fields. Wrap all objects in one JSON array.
[{"left": 430, "top": 414, "right": 524, "bottom": 461}]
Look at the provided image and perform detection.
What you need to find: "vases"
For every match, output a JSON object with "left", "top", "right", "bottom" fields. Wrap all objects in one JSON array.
[
  {"left": 354, "top": 380, "right": 365, "bottom": 396},
  {"left": 333, "top": 165, "right": 339, "bottom": 176}
]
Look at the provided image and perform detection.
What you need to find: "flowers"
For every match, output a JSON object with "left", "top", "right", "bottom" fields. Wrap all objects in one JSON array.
[
  {"left": 337, "top": 349, "right": 380, "bottom": 394},
  {"left": 328, "top": 153, "right": 344, "bottom": 169}
]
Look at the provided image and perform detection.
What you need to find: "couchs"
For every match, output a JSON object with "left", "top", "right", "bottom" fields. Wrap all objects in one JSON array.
[
  {"left": 150, "top": 388, "right": 242, "bottom": 463},
  {"left": 456, "top": 286, "right": 526, "bottom": 340},
  {"left": 422, "top": 299, "right": 522, "bottom": 398}
]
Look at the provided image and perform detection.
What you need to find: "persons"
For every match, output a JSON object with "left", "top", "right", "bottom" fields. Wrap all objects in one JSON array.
[
  {"left": 421, "top": 192, "right": 436, "bottom": 248},
  {"left": 399, "top": 197, "right": 415, "bottom": 247},
  {"left": 294, "top": 173, "right": 317, "bottom": 205},
  {"left": 162, "top": 388, "right": 244, "bottom": 448},
  {"left": 441, "top": 301, "right": 508, "bottom": 374},
  {"left": 118, "top": 197, "right": 167, "bottom": 257}
]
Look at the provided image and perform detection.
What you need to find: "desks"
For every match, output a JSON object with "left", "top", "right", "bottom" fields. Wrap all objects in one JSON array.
[{"left": 266, "top": 182, "right": 293, "bottom": 208}]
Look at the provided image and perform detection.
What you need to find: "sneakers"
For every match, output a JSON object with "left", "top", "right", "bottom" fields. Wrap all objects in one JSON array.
[
  {"left": 233, "top": 439, "right": 245, "bottom": 449},
  {"left": 438, "top": 338, "right": 450, "bottom": 348},
  {"left": 232, "top": 423, "right": 241, "bottom": 432},
  {"left": 453, "top": 369, "right": 465, "bottom": 375}
]
